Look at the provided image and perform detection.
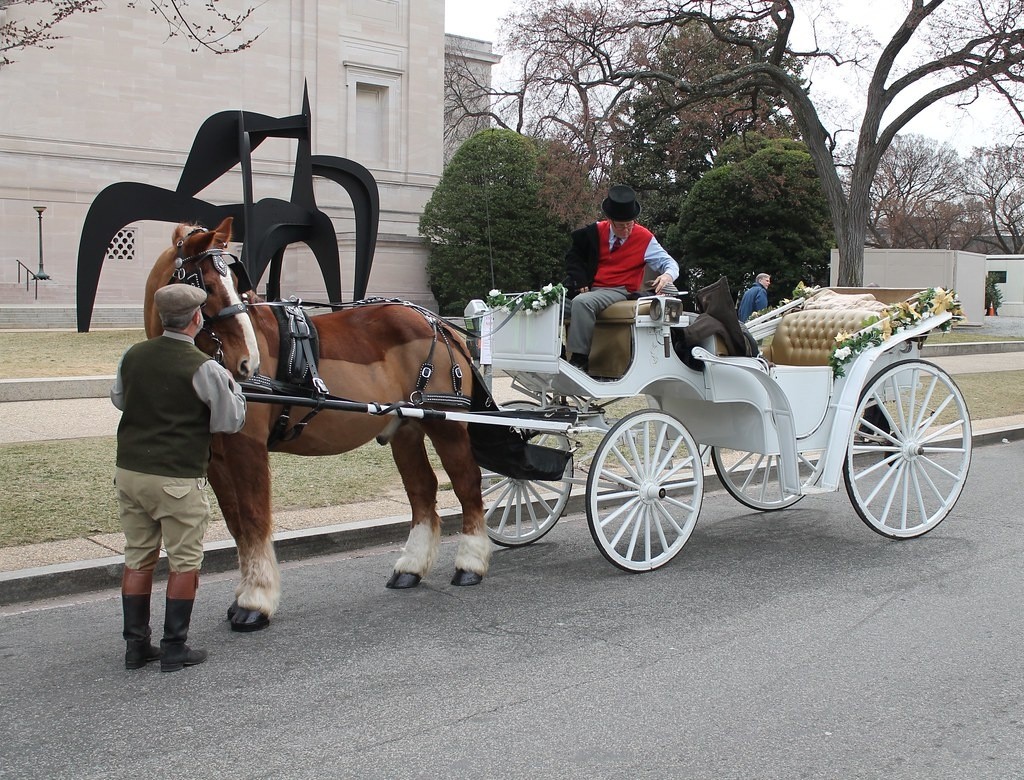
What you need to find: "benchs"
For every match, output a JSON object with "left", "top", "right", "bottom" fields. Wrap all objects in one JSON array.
[
  {"left": 683, "top": 309, "right": 835, "bottom": 436},
  {"left": 561, "top": 297, "right": 656, "bottom": 374}
]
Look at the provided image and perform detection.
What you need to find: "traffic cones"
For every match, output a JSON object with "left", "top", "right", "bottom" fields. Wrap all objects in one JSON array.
[{"left": 989, "top": 301, "right": 994, "bottom": 316}]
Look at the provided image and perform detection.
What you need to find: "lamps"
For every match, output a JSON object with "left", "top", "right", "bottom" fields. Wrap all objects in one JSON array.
[{"left": 31, "top": 206, "right": 51, "bottom": 280}]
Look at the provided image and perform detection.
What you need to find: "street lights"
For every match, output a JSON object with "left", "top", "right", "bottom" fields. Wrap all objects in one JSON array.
[{"left": 32, "top": 206, "right": 50, "bottom": 280}]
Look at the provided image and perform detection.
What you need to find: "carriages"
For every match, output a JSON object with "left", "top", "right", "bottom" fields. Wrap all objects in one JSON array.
[{"left": 140, "top": 216, "right": 973, "bottom": 633}]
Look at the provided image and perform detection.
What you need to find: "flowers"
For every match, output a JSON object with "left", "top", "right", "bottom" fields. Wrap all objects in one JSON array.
[
  {"left": 826, "top": 287, "right": 965, "bottom": 377},
  {"left": 742, "top": 286, "right": 813, "bottom": 324},
  {"left": 487, "top": 282, "right": 570, "bottom": 315}
]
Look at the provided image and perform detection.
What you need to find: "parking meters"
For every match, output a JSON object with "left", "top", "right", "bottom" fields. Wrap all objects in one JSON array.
[{"left": 463, "top": 300, "right": 490, "bottom": 370}]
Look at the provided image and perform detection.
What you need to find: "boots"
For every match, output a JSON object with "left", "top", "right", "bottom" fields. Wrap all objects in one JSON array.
[
  {"left": 159, "top": 572, "right": 208, "bottom": 672},
  {"left": 121, "top": 565, "right": 158, "bottom": 670}
]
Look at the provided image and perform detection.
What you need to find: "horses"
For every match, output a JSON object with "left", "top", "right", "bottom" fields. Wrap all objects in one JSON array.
[{"left": 141, "top": 214, "right": 492, "bottom": 632}]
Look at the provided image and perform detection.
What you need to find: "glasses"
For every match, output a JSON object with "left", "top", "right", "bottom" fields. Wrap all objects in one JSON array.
[{"left": 611, "top": 221, "right": 636, "bottom": 231}]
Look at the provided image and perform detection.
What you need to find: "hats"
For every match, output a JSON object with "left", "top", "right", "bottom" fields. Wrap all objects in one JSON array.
[
  {"left": 602, "top": 185, "right": 641, "bottom": 222},
  {"left": 154, "top": 283, "right": 208, "bottom": 315}
]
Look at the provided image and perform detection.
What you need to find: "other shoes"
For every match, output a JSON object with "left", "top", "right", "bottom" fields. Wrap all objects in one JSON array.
[{"left": 568, "top": 359, "right": 589, "bottom": 375}]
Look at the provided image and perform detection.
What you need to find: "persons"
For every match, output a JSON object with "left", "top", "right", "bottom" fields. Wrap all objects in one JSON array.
[
  {"left": 111, "top": 283, "right": 247, "bottom": 671},
  {"left": 738, "top": 273, "right": 770, "bottom": 345},
  {"left": 853, "top": 283, "right": 890, "bottom": 442},
  {"left": 560, "top": 185, "right": 679, "bottom": 375}
]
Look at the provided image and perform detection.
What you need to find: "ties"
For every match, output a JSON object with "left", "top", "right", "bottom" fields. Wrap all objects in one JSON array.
[{"left": 610, "top": 237, "right": 621, "bottom": 253}]
